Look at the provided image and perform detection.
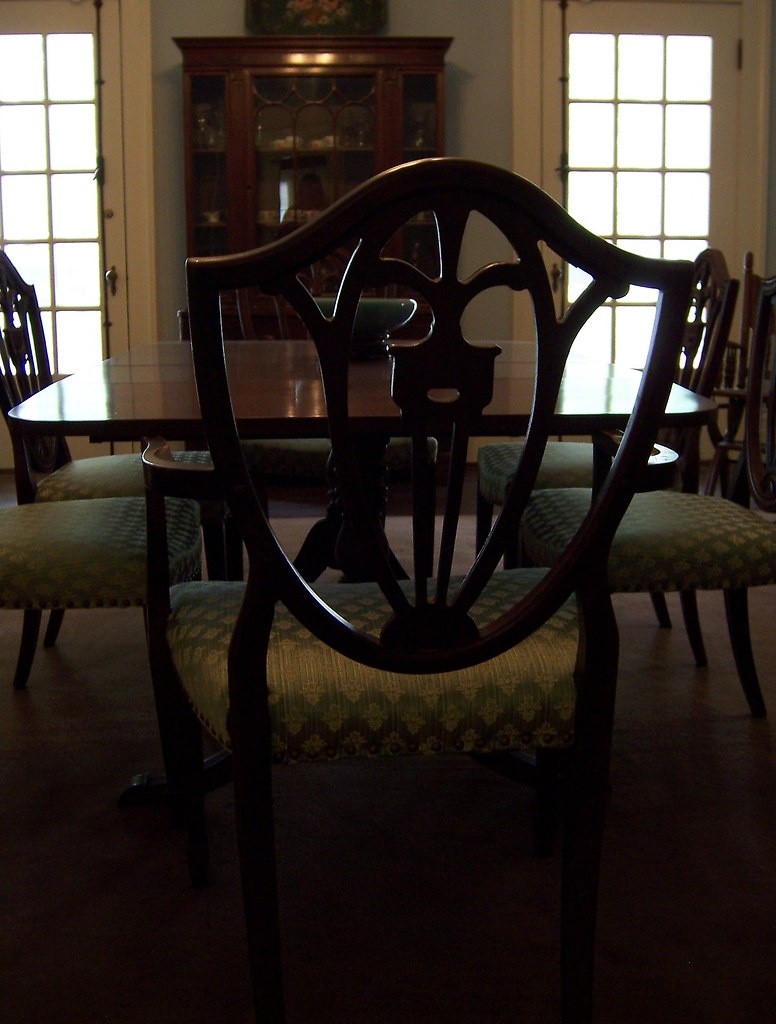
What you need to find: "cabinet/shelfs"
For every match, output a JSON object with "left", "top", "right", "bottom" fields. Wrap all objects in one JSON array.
[{"left": 173, "top": 33, "right": 453, "bottom": 341}]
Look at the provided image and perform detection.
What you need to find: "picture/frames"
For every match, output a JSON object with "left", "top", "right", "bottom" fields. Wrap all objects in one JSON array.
[{"left": 243, "top": 0, "right": 388, "bottom": 32}]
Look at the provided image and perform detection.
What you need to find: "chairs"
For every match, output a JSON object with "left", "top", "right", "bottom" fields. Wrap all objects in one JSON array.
[{"left": 0, "top": 161, "right": 776, "bottom": 1024}]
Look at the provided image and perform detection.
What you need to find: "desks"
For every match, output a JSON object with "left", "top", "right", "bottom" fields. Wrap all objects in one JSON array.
[{"left": 7, "top": 341, "right": 720, "bottom": 796}]
